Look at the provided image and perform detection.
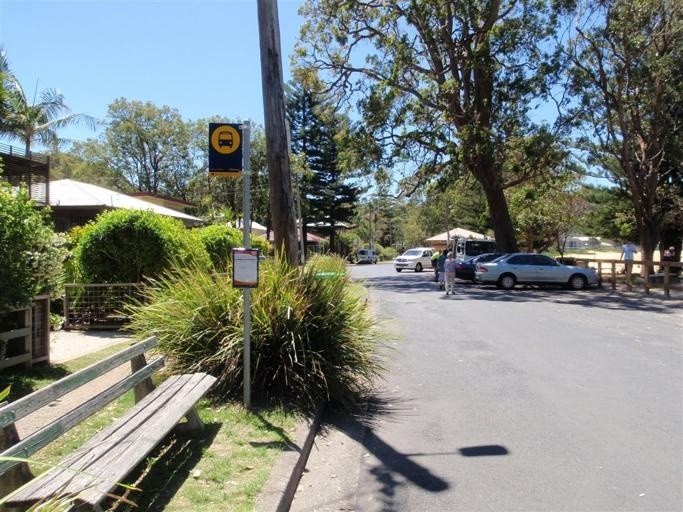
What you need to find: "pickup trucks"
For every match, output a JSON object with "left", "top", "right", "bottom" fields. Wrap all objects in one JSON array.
[{"left": 393, "top": 246, "right": 438, "bottom": 274}]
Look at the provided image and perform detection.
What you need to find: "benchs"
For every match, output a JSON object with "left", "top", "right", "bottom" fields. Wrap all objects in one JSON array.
[{"left": 0, "top": 335, "right": 220, "bottom": 512}]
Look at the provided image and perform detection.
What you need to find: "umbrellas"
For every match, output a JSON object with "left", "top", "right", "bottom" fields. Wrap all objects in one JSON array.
[
  {"left": 223, "top": 217, "right": 271, "bottom": 236},
  {"left": 423, "top": 226, "right": 492, "bottom": 244},
  {"left": 257, "top": 228, "right": 320, "bottom": 243}
]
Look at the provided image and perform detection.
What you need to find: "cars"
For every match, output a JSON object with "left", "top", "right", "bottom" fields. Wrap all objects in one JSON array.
[
  {"left": 474, "top": 253, "right": 598, "bottom": 290},
  {"left": 453, "top": 252, "right": 505, "bottom": 285}
]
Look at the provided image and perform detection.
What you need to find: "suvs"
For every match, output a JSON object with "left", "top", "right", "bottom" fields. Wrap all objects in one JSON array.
[{"left": 355, "top": 248, "right": 377, "bottom": 265}]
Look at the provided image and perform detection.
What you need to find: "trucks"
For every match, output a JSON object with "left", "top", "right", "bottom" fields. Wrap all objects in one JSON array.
[{"left": 447, "top": 233, "right": 498, "bottom": 265}]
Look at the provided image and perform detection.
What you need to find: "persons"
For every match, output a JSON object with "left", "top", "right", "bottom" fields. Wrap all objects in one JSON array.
[
  {"left": 663, "top": 246, "right": 676, "bottom": 260},
  {"left": 619, "top": 237, "right": 638, "bottom": 273},
  {"left": 443, "top": 251, "right": 456, "bottom": 296},
  {"left": 437, "top": 249, "right": 448, "bottom": 290},
  {"left": 430, "top": 247, "right": 442, "bottom": 275}
]
